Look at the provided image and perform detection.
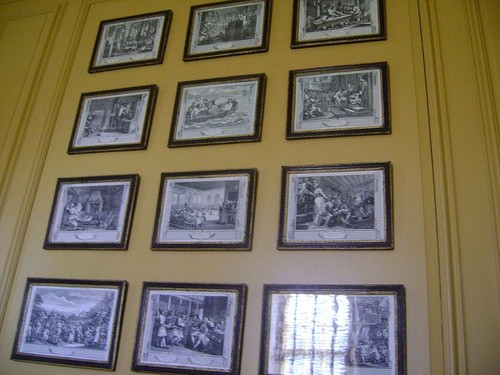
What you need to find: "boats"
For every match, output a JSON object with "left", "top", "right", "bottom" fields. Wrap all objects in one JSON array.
[{"left": 186, "top": 99, "right": 239, "bottom": 122}]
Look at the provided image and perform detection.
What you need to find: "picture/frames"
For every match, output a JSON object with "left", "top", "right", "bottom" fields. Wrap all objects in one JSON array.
[
  {"left": 132, "top": 278, "right": 248, "bottom": 374},
  {"left": 42, "top": 174, "right": 141, "bottom": 252},
  {"left": 285, "top": 60, "right": 391, "bottom": 141},
  {"left": 260, "top": 283, "right": 409, "bottom": 375},
  {"left": 182, "top": 0, "right": 274, "bottom": 62},
  {"left": 86, "top": 8, "right": 172, "bottom": 75},
  {"left": 279, "top": 160, "right": 394, "bottom": 249},
  {"left": 289, "top": 1, "right": 387, "bottom": 49},
  {"left": 9, "top": 278, "right": 128, "bottom": 373},
  {"left": 66, "top": 83, "right": 159, "bottom": 155},
  {"left": 166, "top": 72, "right": 266, "bottom": 148},
  {"left": 149, "top": 168, "right": 259, "bottom": 251}
]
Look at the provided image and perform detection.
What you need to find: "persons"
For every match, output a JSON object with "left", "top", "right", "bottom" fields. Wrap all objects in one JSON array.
[
  {"left": 326, "top": 5, "right": 364, "bottom": 25},
  {"left": 300, "top": 75, "right": 369, "bottom": 119},
  {"left": 28, "top": 306, "right": 110, "bottom": 348},
  {"left": 105, "top": 24, "right": 155, "bottom": 56},
  {"left": 188, "top": 98, "right": 219, "bottom": 119},
  {"left": 299, "top": 181, "right": 374, "bottom": 226},
  {"left": 86, "top": 98, "right": 131, "bottom": 135},
  {"left": 176, "top": 196, "right": 236, "bottom": 228},
  {"left": 152, "top": 307, "right": 225, "bottom": 355},
  {"left": 199, "top": 14, "right": 251, "bottom": 42},
  {"left": 65, "top": 199, "right": 117, "bottom": 228}
]
